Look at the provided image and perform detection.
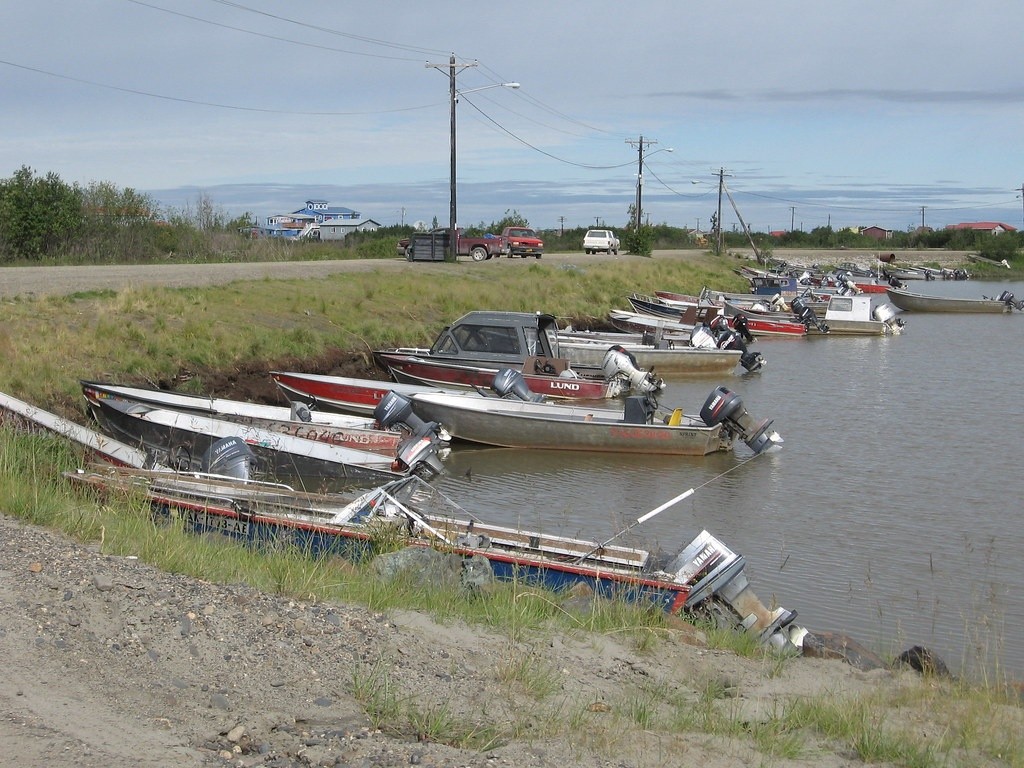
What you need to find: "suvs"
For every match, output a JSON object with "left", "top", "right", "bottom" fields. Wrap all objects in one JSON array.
[{"left": 582, "top": 229, "right": 621, "bottom": 257}]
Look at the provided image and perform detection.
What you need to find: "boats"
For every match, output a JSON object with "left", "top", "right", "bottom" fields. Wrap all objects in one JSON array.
[
  {"left": 374, "top": 346, "right": 669, "bottom": 404},
  {"left": 384, "top": 310, "right": 767, "bottom": 382},
  {"left": 59, "top": 461, "right": 823, "bottom": 660},
  {"left": 0, "top": 390, "right": 259, "bottom": 488},
  {"left": 884, "top": 285, "right": 1024, "bottom": 320},
  {"left": 265, "top": 368, "right": 785, "bottom": 458},
  {"left": 597, "top": 253, "right": 973, "bottom": 356},
  {"left": 77, "top": 376, "right": 456, "bottom": 489}
]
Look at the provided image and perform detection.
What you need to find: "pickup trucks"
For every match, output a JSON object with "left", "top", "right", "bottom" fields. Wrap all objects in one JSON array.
[
  {"left": 492, "top": 227, "right": 544, "bottom": 260},
  {"left": 397, "top": 227, "right": 510, "bottom": 261}
]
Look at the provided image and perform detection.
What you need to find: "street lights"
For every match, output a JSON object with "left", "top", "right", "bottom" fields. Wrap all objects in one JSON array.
[
  {"left": 637, "top": 148, "right": 672, "bottom": 232},
  {"left": 692, "top": 179, "right": 724, "bottom": 255},
  {"left": 448, "top": 82, "right": 521, "bottom": 262}
]
